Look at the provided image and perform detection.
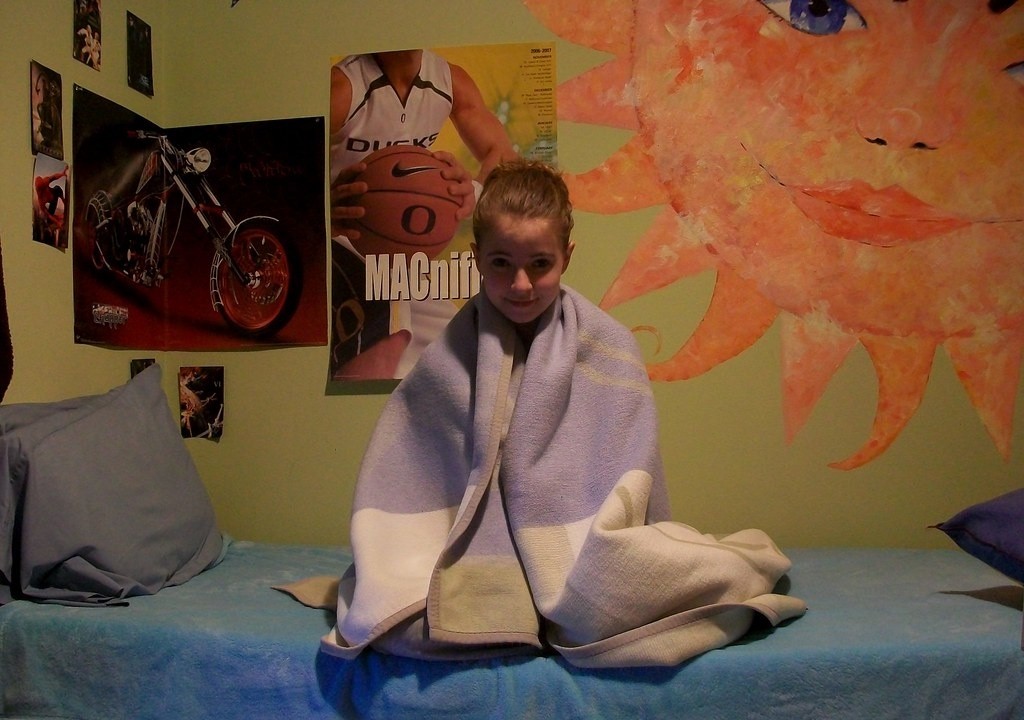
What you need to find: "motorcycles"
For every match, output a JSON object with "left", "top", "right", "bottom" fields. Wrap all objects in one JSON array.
[{"left": 83, "top": 130, "right": 302, "bottom": 338}]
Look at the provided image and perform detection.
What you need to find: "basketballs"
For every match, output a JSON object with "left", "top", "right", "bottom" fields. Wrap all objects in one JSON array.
[{"left": 340, "top": 146, "right": 465, "bottom": 269}]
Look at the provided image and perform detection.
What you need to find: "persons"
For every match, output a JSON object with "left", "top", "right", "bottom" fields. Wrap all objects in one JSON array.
[
  {"left": 35, "top": 163, "right": 69, "bottom": 229},
  {"left": 330, "top": 50, "right": 525, "bottom": 380},
  {"left": 268, "top": 166, "right": 808, "bottom": 671},
  {"left": 76, "top": 25, "right": 102, "bottom": 71}
]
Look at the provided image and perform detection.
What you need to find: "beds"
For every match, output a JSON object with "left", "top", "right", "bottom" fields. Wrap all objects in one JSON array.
[{"left": 1, "top": 538, "right": 1024, "bottom": 719}]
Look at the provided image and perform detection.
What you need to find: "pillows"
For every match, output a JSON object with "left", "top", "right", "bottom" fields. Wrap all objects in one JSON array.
[
  {"left": 0, "top": 363, "right": 225, "bottom": 608},
  {"left": 927, "top": 487, "right": 1024, "bottom": 585}
]
